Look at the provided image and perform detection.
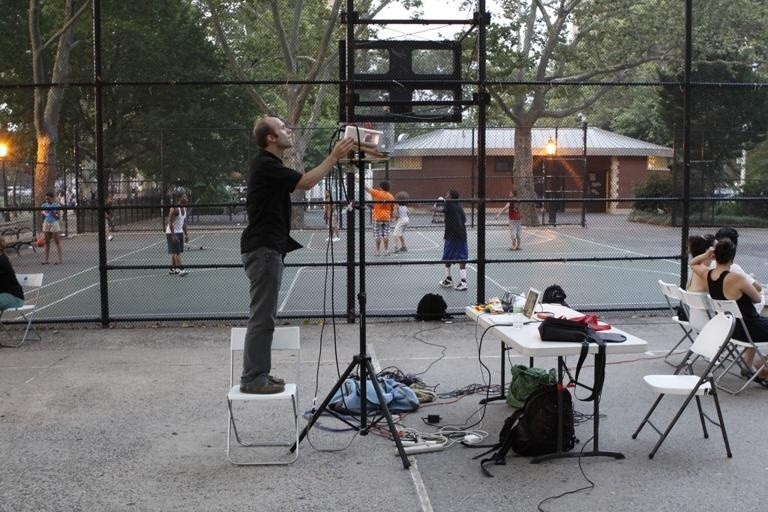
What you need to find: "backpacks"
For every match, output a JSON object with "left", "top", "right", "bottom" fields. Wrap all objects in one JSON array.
[{"left": 499, "top": 383, "right": 576, "bottom": 458}]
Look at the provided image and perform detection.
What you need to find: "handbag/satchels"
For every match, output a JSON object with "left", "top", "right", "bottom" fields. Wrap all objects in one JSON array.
[
  {"left": 537, "top": 316, "right": 597, "bottom": 343},
  {"left": 506, "top": 364, "right": 556, "bottom": 410}
]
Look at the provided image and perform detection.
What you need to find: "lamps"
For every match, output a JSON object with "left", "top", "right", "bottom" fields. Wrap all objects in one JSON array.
[{"left": 546, "top": 135, "right": 558, "bottom": 156}]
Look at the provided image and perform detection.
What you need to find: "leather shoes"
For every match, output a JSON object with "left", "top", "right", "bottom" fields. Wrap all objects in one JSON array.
[
  {"left": 239, "top": 383, "right": 285, "bottom": 395},
  {"left": 269, "top": 376, "right": 286, "bottom": 384}
]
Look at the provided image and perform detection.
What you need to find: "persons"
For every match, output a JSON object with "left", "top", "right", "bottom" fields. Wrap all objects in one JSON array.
[
  {"left": 104, "top": 197, "right": 112, "bottom": 241},
  {"left": 393, "top": 191, "right": 411, "bottom": 253},
  {"left": 166, "top": 192, "right": 189, "bottom": 277},
  {"left": 495, "top": 189, "right": 522, "bottom": 250},
  {"left": 323, "top": 189, "right": 340, "bottom": 242},
  {"left": 688, "top": 227, "right": 768, "bottom": 385},
  {"left": 432, "top": 196, "right": 445, "bottom": 221},
  {"left": 40, "top": 192, "right": 62, "bottom": 265},
  {"left": 441, "top": 188, "right": 469, "bottom": 291},
  {"left": 240, "top": 115, "right": 354, "bottom": 394},
  {"left": 0, "top": 237, "right": 24, "bottom": 311},
  {"left": 363, "top": 181, "right": 399, "bottom": 255}
]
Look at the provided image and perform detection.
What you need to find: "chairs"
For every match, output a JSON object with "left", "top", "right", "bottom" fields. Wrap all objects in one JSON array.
[
  {"left": 632, "top": 311, "right": 735, "bottom": 458},
  {"left": 703, "top": 293, "right": 767, "bottom": 396},
  {"left": 677, "top": 287, "right": 749, "bottom": 382},
  {"left": 225, "top": 327, "right": 300, "bottom": 467},
  {"left": 0, "top": 273, "right": 44, "bottom": 348},
  {"left": 657, "top": 279, "right": 717, "bottom": 370}
]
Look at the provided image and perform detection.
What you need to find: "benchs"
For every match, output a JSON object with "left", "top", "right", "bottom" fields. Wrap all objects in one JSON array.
[{"left": 1, "top": 227, "right": 36, "bottom": 255}]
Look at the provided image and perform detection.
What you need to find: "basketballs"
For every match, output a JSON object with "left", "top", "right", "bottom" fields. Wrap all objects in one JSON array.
[
  {"left": 36, "top": 239, "right": 45, "bottom": 247},
  {"left": 395, "top": 190, "right": 410, "bottom": 204}
]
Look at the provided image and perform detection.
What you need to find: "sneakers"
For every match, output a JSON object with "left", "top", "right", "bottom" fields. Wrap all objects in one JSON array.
[
  {"left": 454, "top": 279, "right": 469, "bottom": 291},
  {"left": 178, "top": 269, "right": 189, "bottom": 277},
  {"left": 439, "top": 276, "right": 454, "bottom": 287},
  {"left": 168, "top": 268, "right": 180, "bottom": 275}
]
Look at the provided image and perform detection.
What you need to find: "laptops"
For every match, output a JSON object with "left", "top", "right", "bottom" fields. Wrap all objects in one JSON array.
[{"left": 488, "top": 287, "right": 541, "bottom": 325}]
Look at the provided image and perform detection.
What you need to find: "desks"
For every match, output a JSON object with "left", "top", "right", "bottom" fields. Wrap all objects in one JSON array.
[{"left": 466, "top": 301, "right": 648, "bottom": 465}]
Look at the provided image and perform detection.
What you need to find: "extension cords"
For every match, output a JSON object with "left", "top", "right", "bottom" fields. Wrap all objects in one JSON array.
[
  {"left": 401, "top": 440, "right": 436, "bottom": 447},
  {"left": 394, "top": 443, "right": 443, "bottom": 456}
]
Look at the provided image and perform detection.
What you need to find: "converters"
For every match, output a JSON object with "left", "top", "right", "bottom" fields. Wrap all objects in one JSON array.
[
  {"left": 428, "top": 415, "right": 440, "bottom": 423},
  {"left": 465, "top": 435, "right": 480, "bottom": 443}
]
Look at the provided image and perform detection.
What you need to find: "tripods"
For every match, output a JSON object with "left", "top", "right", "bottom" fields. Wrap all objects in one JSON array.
[{"left": 290, "top": 164, "right": 411, "bottom": 469}]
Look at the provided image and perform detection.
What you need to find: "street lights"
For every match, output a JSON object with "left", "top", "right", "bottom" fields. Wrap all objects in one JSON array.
[
  {"left": 0, "top": 142, "right": 14, "bottom": 223},
  {"left": 546, "top": 126, "right": 558, "bottom": 224},
  {"left": 577, "top": 113, "right": 588, "bottom": 228}
]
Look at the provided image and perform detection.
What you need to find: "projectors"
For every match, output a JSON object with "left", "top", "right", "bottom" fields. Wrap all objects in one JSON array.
[{"left": 344, "top": 126, "right": 383, "bottom": 147}]
